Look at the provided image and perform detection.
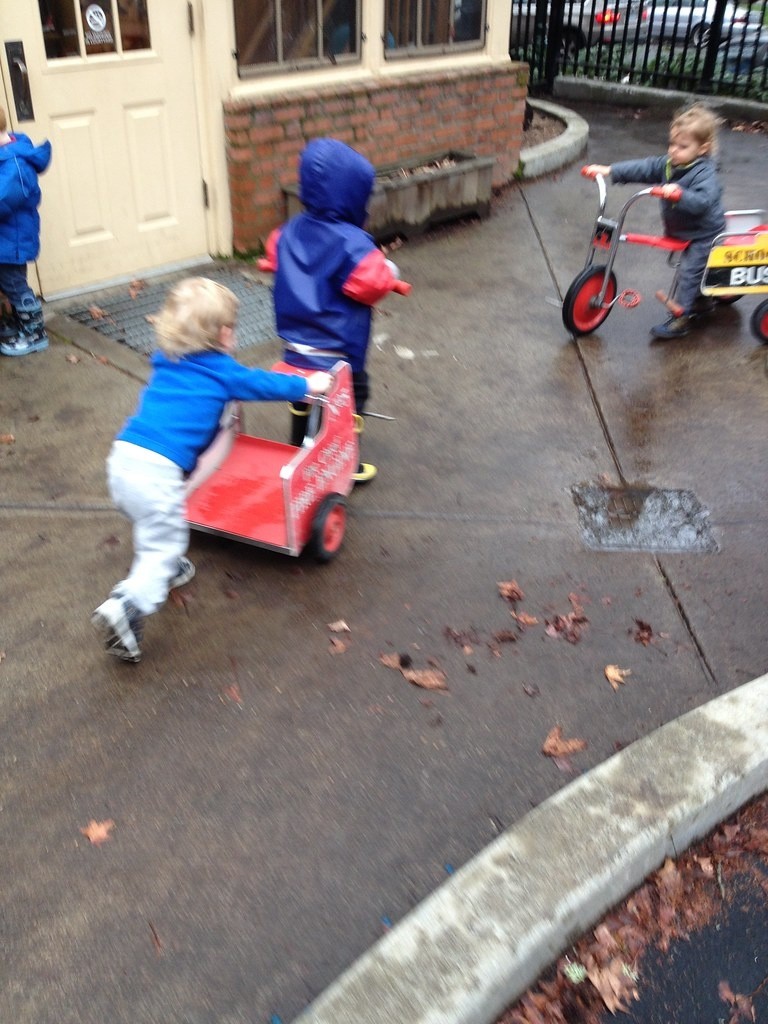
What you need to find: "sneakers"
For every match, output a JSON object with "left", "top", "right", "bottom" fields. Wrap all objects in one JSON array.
[
  {"left": 692, "top": 296, "right": 714, "bottom": 313},
  {"left": 650, "top": 312, "right": 691, "bottom": 337}
]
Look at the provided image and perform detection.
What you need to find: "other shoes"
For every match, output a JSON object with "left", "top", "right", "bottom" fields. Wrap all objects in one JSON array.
[
  {"left": 352, "top": 464, "right": 376, "bottom": 481},
  {"left": 167, "top": 556, "right": 195, "bottom": 588},
  {"left": 91, "top": 597, "right": 143, "bottom": 662}
]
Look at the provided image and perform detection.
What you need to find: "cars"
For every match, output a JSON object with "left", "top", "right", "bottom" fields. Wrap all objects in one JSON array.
[
  {"left": 646, "top": 0, "right": 768, "bottom": 77},
  {"left": 510, "top": 0, "right": 648, "bottom": 61}
]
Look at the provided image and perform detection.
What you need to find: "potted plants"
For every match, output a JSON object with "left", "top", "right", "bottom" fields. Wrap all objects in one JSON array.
[{"left": 283, "top": 147, "right": 497, "bottom": 240}]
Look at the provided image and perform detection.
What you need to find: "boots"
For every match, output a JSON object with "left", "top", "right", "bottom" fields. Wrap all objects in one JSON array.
[
  {"left": 0, "top": 301, "right": 18, "bottom": 336},
  {"left": 0, "top": 300, "right": 49, "bottom": 356}
]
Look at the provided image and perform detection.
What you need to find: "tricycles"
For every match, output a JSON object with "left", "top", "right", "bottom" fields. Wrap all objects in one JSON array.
[{"left": 561, "top": 165, "right": 768, "bottom": 348}]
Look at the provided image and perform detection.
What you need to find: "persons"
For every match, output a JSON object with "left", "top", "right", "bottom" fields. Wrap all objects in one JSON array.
[
  {"left": 88, "top": 277, "right": 333, "bottom": 663},
  {"left": 259, "top": 139, "right": 401, "bottom": 481},
  {"left": 0, "top": 108, "right": 52, "bottom": 355},
  {"left": 586, "top": 107, "right": 728, "bottom": 337}
]
